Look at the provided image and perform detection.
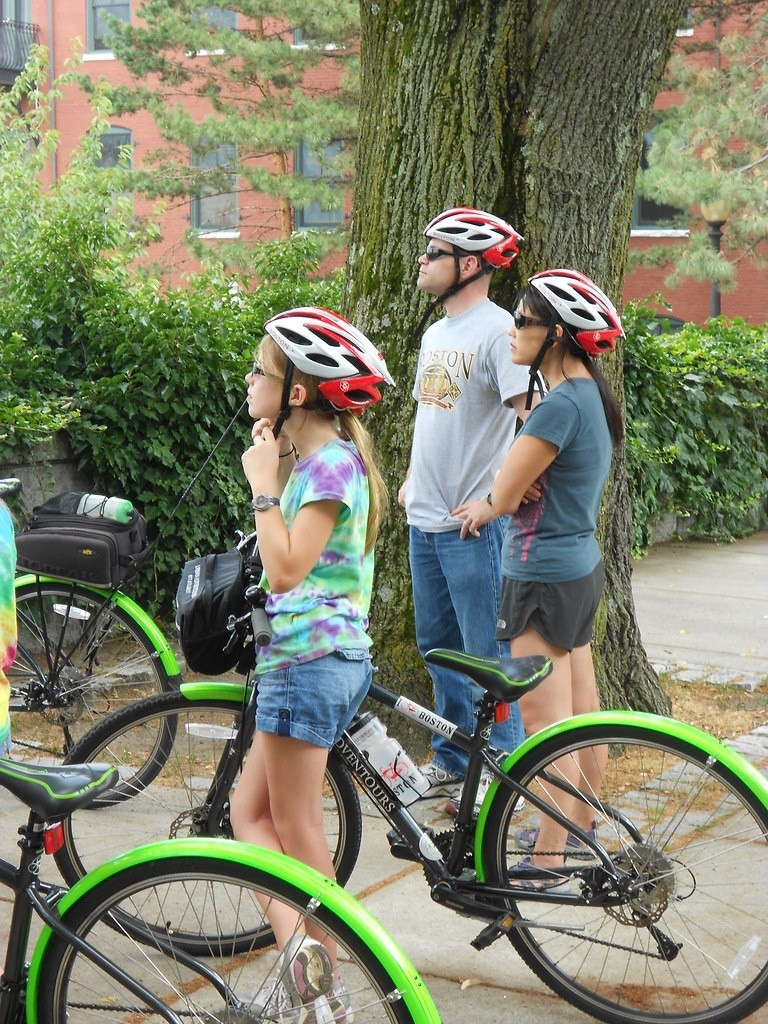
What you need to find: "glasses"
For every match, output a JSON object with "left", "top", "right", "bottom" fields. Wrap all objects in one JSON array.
[
  {"left": 251, "top": 362, "right": 284, "bottom": 382},
  {"left": 425, "top": 246, "right": 479, "bottom": 267},
  {"left": 513, "top": 310, "right": 549, "bottom": 330}
]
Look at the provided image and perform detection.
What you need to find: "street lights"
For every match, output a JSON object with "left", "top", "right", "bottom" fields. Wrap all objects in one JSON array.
[{"left": 701, "top": 191, "right": 730, "bottom": 319}]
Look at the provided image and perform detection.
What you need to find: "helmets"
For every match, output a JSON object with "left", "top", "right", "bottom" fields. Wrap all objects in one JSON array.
[
  {"left": 263, "top": 305, "right": 396, "bottom": 414},
  {"left": 527, "top": 269, "right": 627, "bottom": 358},
  {"left": 422, "top": 207, "right": 525, "bottom": 268}
]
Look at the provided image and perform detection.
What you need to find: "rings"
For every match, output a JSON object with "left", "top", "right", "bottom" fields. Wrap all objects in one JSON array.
[{"left": 464, "top": 521, "right": 470, "bottom": 526}]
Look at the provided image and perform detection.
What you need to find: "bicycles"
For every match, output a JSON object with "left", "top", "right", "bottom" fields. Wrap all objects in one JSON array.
[
  {"left": 0, "top": 761, "right": 447, "bottom": 1024},
  {"left": 48, "top": 527, "right": 767, "bottom": 1024},
  {"left": 0, "top": 478, "right": 177, "bottom": 807}
]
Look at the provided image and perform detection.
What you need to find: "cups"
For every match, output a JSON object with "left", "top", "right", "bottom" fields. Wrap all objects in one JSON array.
[
  {"left": 347, "top": 709, "right": 432, "bottom": 807},
  {"left": 77, "top": 493, "right": 135, "bottom": 524}
]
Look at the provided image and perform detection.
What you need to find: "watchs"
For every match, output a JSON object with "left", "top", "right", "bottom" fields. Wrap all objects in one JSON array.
[{"left": 250, "top": 494, "right": 280, "bottom": 512}]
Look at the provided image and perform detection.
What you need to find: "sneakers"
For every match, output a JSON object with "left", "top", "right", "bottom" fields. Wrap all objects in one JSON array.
[
  {"left": 261, "top": 977, "right": 355, "bottom": 1024},
  {"left": 445, "top": 768, "right": 525, "bottom": 816},
  {"left": 290, "top": 939, "right": 348, "bottom": 1024},
  {"left": 514, "top": 819, "right": 599, "bottom": 861},
  {"left": 508, "top": 856, "right": 570, "bottom": 888},
  {"left": 414, "top": 762, "right": 464, "bottom": 798}
]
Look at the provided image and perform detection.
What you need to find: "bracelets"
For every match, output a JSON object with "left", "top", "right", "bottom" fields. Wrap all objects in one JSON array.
[
  {"left": 279, "top": 449, "right": 294, "bottom": 457},
  {"left": 487, "top": 493, "right": 492, "bottom": 504}
]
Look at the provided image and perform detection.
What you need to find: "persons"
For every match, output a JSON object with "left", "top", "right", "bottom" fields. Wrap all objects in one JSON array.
[
  {"left": 398, "top": 208, "right": 548, "bottom": 817},
  {"left": 489, "top": 269, "right": 626, "bottom": 895},
  {"left": 230, "top": 307, "right": 395, "bottom": 1024}
]
